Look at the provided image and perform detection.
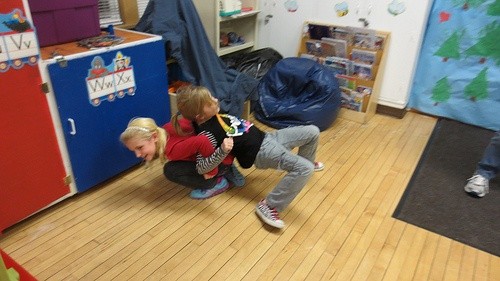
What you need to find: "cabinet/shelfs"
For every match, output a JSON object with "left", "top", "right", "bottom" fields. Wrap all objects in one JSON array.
[
  {"left": 0, "top": 0, "right": 171, "bottom": 233},
  {"left": 192, "top": 0, "right": 262, "bottom": 57},
  {"left": 166, "top": 57, "right": 176, "bottom": 65},
  {"left": 297, "top": 20, "right": 391, "bottom": 127}
]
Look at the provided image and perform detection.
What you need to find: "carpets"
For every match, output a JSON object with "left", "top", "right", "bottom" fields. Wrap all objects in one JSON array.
[{"left": 391, "top": 116, "right": 500, "bottom": 258}]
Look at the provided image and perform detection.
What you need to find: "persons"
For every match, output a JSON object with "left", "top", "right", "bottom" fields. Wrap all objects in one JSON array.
[
  {"left": 172, "top": 85, "right": 324, "bottom": 228},
  {"left": 120, "top": 117, "right": 245, "bottom": 198},
  {"left": 463, "top": 129, "right": 500, "bottom": 197}
]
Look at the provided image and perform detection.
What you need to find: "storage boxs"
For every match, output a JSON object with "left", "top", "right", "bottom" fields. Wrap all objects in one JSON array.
[
  {"left": 241, "top": 100, "right": 250, "bottom": 121},
  {"left": 169, "top": 92, "right": 178, "bottom": 118},
  {"left": 27, "top": 0, "right": 102, "bottom": 49}
]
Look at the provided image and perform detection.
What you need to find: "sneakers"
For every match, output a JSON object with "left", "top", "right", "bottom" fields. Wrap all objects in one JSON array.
[
  {"left": 313, "top": 161, "right": 324, "bottom": 171},
  {"left": 464, "top": 174, "right": 489, "bottom": 197},
  {"left": 190, "top": 176, "right": 229, "bottom": 199},
  {"left": 256, "top": 197, "right": 284, "bottom": 228}
]
